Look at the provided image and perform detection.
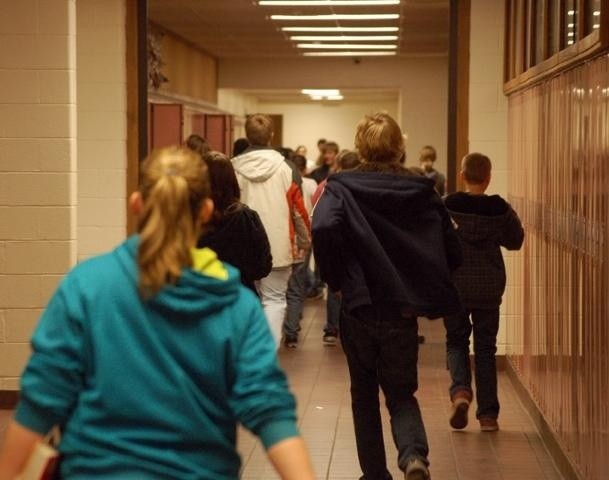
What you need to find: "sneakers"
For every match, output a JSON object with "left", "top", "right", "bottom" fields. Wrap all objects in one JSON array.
[
  {"left": 322, "top": 327, "right": 339, "bottom": 347},
  {"left": 283, "top": 335, "right": 297, "bottom": 347},
  {"left": 448, "top": 389, "right": 469, "bottom": 430},
  {"left": 404, "top": 460, "right": 431, "bottom": 480},
  {"left": 478, "top": 417, "right": 498, "bottom": 433}
]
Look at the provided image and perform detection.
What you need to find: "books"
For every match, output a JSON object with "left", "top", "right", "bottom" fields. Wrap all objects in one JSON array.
[{"left": 20, "top": 441, "right": 59, "bottom": 480}]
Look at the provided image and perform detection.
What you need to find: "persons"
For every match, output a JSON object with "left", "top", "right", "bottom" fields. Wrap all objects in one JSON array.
[
  {"left": 185, "top": 108, "right": 446, "bottom": 347},
  {"left": 443, "top": 151, "right": 523, "bottom": 432},
  {"left": 0, "top": 143, "right": 316, "bottom": 480},
  {"left": 310, "top": 113, "right": 476, "bottom": 480}
]
